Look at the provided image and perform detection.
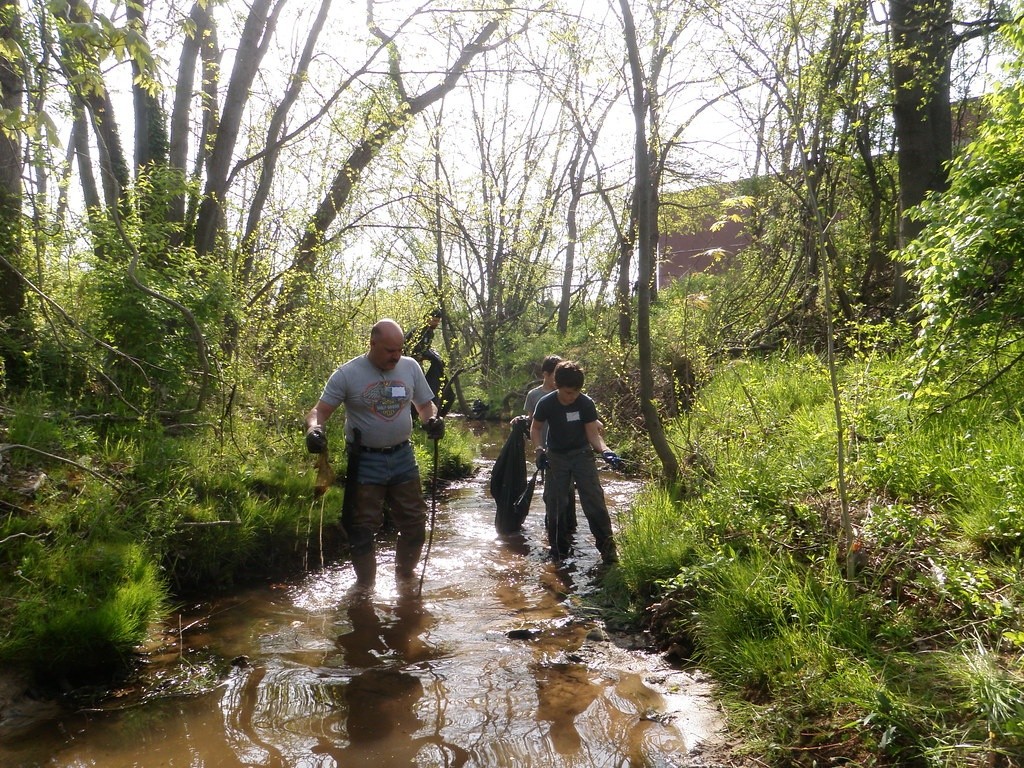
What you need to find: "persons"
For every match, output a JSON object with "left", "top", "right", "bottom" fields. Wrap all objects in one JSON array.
[
  {"left": 510, "top": 355, "right": 624, "bottom": 565},
  {"left": 306, "top": 318, "right": 445, "bottom": 587},
  {"left": 404, "top": 310, "right": 446, "bottom": 403}
]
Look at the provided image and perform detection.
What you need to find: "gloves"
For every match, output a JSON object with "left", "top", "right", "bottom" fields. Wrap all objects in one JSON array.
[
  {"left": 306, "top": 429, "right": 327, "bottom": 453},
  {"left": 423, "top": 415, "right": 446, "bottom": 439},
  {"left": 603, "top": 450, "right": 622, "bottom": 467},
  {"left": 534, "top": 448, "right": 548, "bottom": 470}
]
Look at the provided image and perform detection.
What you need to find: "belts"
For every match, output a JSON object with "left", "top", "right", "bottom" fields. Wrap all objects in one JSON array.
[{"left": 349, "top": 438, "right": 409, "bottom": 455}]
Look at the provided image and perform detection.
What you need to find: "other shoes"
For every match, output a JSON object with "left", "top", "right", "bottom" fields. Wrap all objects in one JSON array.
[{"left": 601, "top": 550, "right": 618, "bottom": 562}]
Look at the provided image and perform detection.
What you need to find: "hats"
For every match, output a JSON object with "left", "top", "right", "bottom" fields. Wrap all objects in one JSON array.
[{"left": 429, "top": 310, "right": 442, "bottom": 319}]
